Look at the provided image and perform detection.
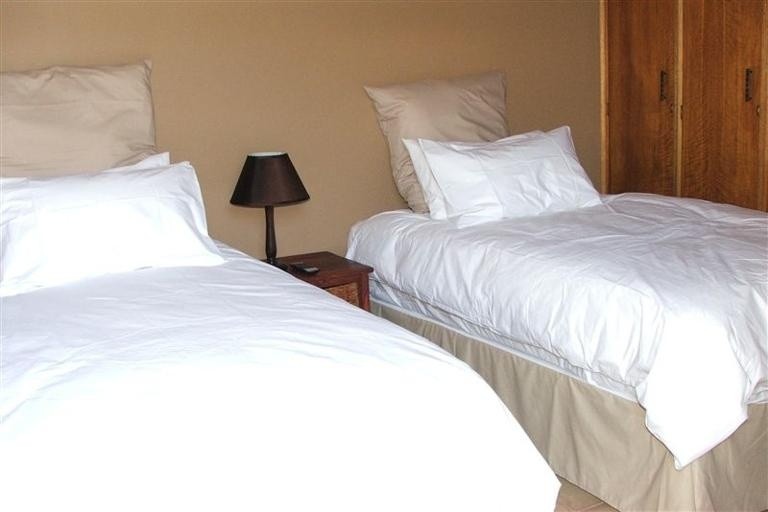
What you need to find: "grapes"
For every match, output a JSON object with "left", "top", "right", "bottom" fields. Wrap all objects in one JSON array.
[{"left": 268, "top": 250, "right": 374, "bottom": 315}]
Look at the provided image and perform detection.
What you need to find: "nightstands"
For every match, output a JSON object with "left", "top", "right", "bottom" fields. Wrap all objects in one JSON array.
[{"left": 268, "top": 250, "right": 374, "bottom": 315}]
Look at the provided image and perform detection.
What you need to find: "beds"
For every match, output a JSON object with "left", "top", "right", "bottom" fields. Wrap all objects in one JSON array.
[
  {"left": 346, "top": 193, "right": 768, "bottom": 511},
  {"left": 0, "top": 238, "right": 562, "bottom": 510}
]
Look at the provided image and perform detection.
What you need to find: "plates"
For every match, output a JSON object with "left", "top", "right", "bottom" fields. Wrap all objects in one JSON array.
[
  {"left": 682, "top": 1, "right": 767, "bottom": 216},
  {"left": 602, "top": 4, "right": 677, "bottom": 190}
]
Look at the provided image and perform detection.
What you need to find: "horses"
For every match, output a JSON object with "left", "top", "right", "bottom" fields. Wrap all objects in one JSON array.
[{"left": 290, "top": 261, "right": 319, "bottom": 274}]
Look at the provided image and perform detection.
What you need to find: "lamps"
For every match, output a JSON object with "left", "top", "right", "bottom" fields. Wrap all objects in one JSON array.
[{"left": 228, "top": 153, "right": 309, "bottom": 260}]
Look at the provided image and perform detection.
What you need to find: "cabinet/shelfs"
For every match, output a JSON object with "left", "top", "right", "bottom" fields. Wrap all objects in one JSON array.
[
  {"left": 602, "top": 4, "right": 677, "bottom": 190},
  {"left": 682, "top": 1, "right": 767, "bottom": 216}
]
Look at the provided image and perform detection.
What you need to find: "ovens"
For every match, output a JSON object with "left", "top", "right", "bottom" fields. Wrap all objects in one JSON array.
[
  {"left": 0, "top": 238, "right": 562, "bottom": 510},
  {"left": 346, "top": 193, "right": 768, "bottom": 511}
]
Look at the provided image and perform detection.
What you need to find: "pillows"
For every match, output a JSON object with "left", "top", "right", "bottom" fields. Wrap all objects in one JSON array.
[
  {"left": 401, "top": 128, "right": 564, "bottom": 219},
  {"left": 1, "top": 60, "right": 158, "bottom": 182},
  {"left": 418, "top": 125, "right": 605, "bottom": 232},
  {"left": 0, "top": 150, "right": 225, "bottom": 298},
  {"left": 362, "top": 75, "right": 534, "bottom": 219}
]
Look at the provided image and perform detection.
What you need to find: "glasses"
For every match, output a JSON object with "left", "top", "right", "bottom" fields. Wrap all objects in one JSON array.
[{"left": 228, "top": 153, "right": 309, "bottom": 260}]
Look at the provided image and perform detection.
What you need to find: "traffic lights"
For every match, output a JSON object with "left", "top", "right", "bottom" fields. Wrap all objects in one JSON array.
[
  {"left": 401, "top": 128, "right": 564, "bottom": 219},
  {"left": 362, "top": 75, "right": 534, "bottom": 219},
  {"left": 418, "top": 125, "right": 605, "bottom": 232}
]
